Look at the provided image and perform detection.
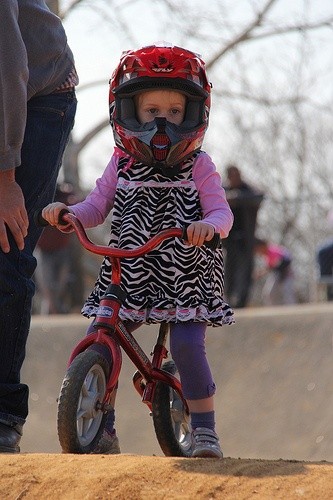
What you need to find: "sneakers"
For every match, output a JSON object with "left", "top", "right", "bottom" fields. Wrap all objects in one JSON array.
[
  {"left": 190, "top": 427, "right": 223, "bottom": 459},
  {"left": 92, "top": 426, "right": 119, "bottom": 454}
]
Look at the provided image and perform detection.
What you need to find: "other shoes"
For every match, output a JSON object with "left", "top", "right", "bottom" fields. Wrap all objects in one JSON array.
[{"left": 0, "top": 425, "right": 22, "bottom": 453}]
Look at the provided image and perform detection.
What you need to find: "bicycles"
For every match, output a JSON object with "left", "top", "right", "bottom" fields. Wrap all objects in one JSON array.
[{"left": 32, "top": 207, "right": 222, "bottom": 456}]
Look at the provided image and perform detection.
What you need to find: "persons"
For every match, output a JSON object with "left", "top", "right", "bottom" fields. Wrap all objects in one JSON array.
[
  {"left": 42, "top": 41, "right": 235, "bottom": 458},
  {"left": 317, "top": 240, "right": 333, "bottom": 302},
  {"left": 221, "top": 167, "right": 257, "bottom": 307},
  {"left": 0, "top": 0, "right": 79, "bottom": 454},
  {"left": 38, "top": 183, "right": 86, "bottom": 314},
  {"left": 255, "top": 239, "right": 294, "bottom": 305}
]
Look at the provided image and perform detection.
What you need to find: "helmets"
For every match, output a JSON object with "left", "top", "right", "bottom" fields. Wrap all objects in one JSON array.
[{"left": 110, "top": 45, "right": 210, "bottom": 166}]
